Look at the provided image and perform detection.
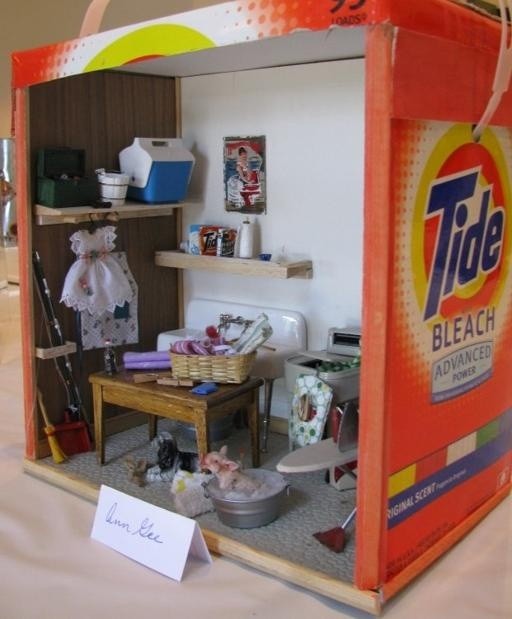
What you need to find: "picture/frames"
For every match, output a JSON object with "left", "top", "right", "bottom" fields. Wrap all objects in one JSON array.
[{"left": 222, "top": 135, "right": 267, "bottom": 216}]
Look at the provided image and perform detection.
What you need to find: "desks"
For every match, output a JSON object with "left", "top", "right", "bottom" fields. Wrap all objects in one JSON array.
[{"left": 88, "top": 364, "right": 265, "bottom": 475}]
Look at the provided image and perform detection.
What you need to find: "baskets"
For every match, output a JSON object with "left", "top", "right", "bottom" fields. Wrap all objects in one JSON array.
[
  {"left": 95, "top": 168, "right": 130, "bottom": 205},
  {"left": 168, "top": 348, "right": 259, "bottom": 384}
]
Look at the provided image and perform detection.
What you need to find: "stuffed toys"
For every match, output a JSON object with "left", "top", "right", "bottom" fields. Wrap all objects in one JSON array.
[{"left": 128, "top": 431, "right": 261, "bottom": 519}]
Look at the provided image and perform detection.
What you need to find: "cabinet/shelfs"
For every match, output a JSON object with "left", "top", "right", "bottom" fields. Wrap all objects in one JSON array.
[
  {"left": 31, "top": 196, "right": 190, "bottom": 225},
  {"left": 154, "top": 248, "right": 313, "bottom": 280}
]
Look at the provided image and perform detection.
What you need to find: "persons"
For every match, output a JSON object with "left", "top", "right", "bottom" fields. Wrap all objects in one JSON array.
[{"left": 227, "top": 147, "right": 256, "bottom": 210}]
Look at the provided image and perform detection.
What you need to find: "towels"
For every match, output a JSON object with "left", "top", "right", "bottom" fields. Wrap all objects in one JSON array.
[{"left": 122, "top": 350, "right": 170, "bottom": 370}]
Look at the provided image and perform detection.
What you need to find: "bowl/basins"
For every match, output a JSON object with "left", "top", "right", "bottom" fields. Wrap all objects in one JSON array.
[
  {"left": 181, "top": 422, "right": 234, "bottom": 442},
  {"left": 201, "top": 469, "right": 290, "bottom": 529}
]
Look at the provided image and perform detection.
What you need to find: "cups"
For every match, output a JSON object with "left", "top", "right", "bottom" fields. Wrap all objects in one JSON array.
[{"left": 258, "top": 253, "right": 271, "bottom": 261}]
